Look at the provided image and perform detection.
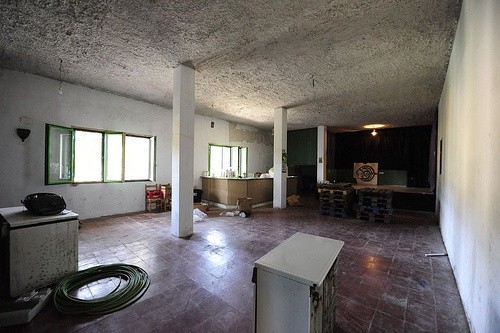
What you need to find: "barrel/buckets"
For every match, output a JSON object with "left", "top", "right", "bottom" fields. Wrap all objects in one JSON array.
[
  {"left": 237, "top": 197, "right": 253, "bottom": 217},
  {"left": 193, "top": 188, "right": 203, "bottom": 203}
]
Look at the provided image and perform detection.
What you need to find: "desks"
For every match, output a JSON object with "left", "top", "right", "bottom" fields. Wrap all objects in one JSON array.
[
  {"left": 252, "top": 233, "right": 344, "bottom": 333},
  {"left": 200, "top": 175, "right": 299, "bottom": 207},
  {"left": 0, "top": 207, "right": 80, "bottom": 298}
]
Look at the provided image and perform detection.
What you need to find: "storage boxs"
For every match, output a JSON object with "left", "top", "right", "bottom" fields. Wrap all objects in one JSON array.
[{"left": 146, "top": 185, "right": 172, "bottom": 199}]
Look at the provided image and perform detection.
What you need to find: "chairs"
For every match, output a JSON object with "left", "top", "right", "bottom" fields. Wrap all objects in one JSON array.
[
  {"left": 158, "top": 183, "right": 171, "bottom": 211},
  {"left": 144, "top": 183, "right": 158, "bottom": 212}
]
